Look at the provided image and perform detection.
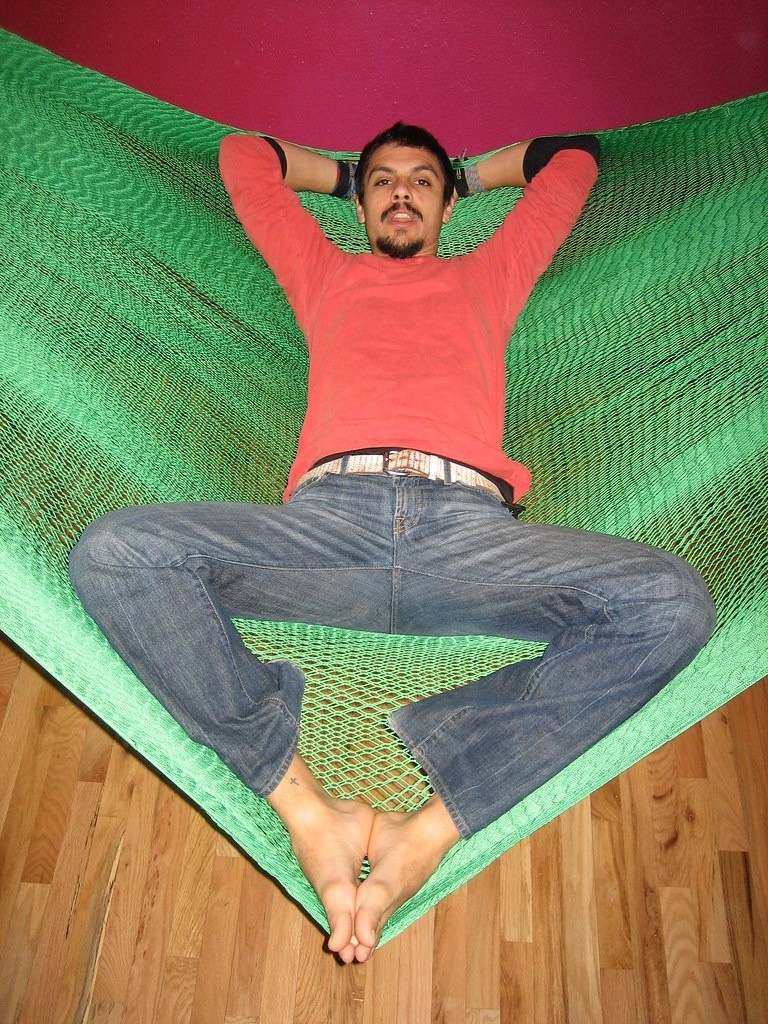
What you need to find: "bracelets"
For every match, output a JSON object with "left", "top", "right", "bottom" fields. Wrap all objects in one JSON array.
[
  {"left": 330, "top": 160, "right": 359, "bottom": 201},
  {"left": 451, "top": 155, "right": 484, "bottom": 199}
]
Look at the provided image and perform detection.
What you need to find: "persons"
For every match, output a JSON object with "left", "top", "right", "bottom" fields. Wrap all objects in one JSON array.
[{"left": 69, "top": 121, "right": 716, "bottom": 963}]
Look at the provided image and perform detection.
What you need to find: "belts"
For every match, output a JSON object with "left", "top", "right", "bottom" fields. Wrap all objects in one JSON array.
[{"left": 295, "top": 449, "right": 506, "bottom": 503}]
